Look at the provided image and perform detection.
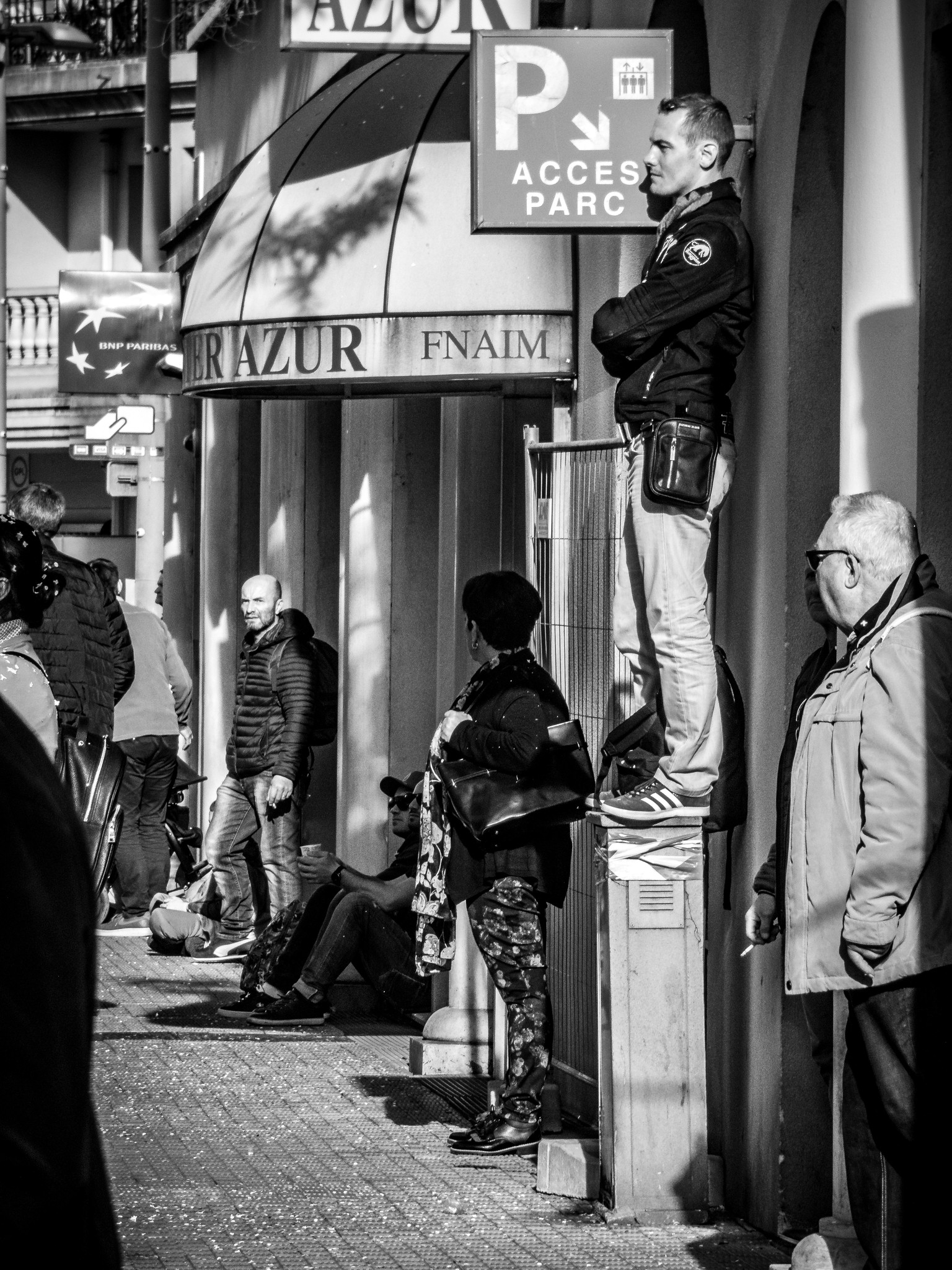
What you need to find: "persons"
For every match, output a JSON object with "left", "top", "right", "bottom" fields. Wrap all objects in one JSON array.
[
  {"left": 18, "top": 483, "right": 135, "bottom": 742},
  {"left": 87, "top": 558, "right": 194, "bottom": 936},
  {"left": 217, "top": 768, "right": 430, "bottom": 1023},
  {"left": 0, "top": 515, "right": 122, "bottom": 1269},
  {"left": 186, "top": 575, "right": 314, "bottom": 960},
  {"left": 748, "top": 490, "right": 952, "bottom": 1270},
  {"left": 584, "top": 91, "right": 757, "bottom": 828},
  {"left": 438, "top": 570, "right": 574, "bottom": 1151}
]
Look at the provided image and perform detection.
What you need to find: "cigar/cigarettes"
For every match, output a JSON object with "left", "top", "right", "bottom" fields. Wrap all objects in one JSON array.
[
  {"left": 740, "top": 942, "right": 756, "bottom": 956},
  {"left": 272, "top": 803, "right": 276, "bottom": 809}
]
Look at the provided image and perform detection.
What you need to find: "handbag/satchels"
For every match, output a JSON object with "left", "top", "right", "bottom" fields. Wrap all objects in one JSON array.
[
  {"left": 648, "top": 416, "right": 719, "bottom": 507},
  {"left": 436, "top": 668, "right": 595, "bottom": 842}
]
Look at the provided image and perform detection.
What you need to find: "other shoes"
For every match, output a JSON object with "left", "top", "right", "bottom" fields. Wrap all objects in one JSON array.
[
  {"left": 95, "top": 913, "right": 153, "bottom": 937},
  {"left": 192, "top": 937, "right": 257, "bottom": 963}
]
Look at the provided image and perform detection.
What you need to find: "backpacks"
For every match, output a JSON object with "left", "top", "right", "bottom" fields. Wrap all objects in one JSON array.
[
  {"left": 0, "top": 651, "right": 127, "bottom": 901},
  {"left": 600, "top": 644, "right": 748, "bottom": 834},
  {"left": 271, "top": 635, "right": 338, "bottom": 746}
]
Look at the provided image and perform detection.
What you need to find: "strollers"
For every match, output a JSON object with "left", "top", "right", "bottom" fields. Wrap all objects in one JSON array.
[{"left": 96, "top": 758, "right": 209, "bottom": 924}]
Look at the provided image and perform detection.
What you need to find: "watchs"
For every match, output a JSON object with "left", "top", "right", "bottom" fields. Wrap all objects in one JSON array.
[{"left": 331, "top": 865, "right": 346, "bottom": 887}]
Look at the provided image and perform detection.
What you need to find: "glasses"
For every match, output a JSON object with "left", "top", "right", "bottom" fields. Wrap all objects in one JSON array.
[
  {"left": 405, "top": 793, "right": 423, "bottom": 806},
  {"left": 388, "top": 795, "right": 409, "bottom": 811},
  {"left": 805, "top": 549, "right": 859, "bottom": 571}
]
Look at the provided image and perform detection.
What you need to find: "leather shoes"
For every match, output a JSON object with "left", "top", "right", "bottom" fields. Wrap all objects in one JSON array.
[
  {"left": 448, "top": 1104, "right": 502, "bottom": 1145},
  {"left": 450, "top": 1120, "right": 543, "bottom": 1160}
]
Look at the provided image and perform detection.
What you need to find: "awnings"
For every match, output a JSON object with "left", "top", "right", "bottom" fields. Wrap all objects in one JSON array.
[{"left": 180, "top": 51, "right": 570, "bottom": 402}]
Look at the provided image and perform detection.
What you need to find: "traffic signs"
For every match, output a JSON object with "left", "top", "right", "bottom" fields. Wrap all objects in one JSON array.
[{"left": 467, "top": 28, "right": 678, "bottom": 233}]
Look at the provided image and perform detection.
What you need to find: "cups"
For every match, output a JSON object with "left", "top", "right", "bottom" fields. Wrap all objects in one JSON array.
[{"left": 300, "top": 844, "right": 322, "bottom": 858}]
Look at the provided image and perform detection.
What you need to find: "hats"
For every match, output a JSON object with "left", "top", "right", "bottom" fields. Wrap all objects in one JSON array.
[{"left": 380, "top": 771, "right": 425, "bottom": 797}]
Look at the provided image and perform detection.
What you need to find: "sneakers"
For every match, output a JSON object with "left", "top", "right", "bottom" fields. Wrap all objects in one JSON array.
[
  {"left": 585, "top": 776, "right": 649, "bottom": 809},
  {"left": 218, "top": 988, "right": 283, "bottom": 1018},
  {"left": 601, "top": 777, "right": 710, "bottom": 820},
  {"left": 246, "top": 988, "right": 325, "bottom": 1025}
]
